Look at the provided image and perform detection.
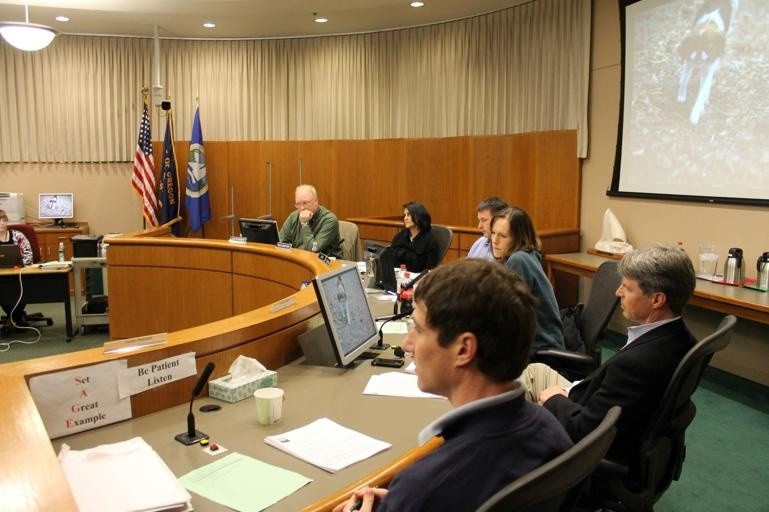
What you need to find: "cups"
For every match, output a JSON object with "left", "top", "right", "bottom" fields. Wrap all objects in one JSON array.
[
  {"left": 698, "top": 244, "right": 719, "bottom": 277},
  {"left": 255, "top": 388, "right": 284, "bottom": 426}
]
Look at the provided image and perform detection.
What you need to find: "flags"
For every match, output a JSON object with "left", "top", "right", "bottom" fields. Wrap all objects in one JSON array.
[
  {"left": 158, "top": 110, "right": 181, "bottom": 237},
  {"left": 131, "top": 99, "right": 158, "bottom": 230},
  {"left": 185, "top": 107, "right": 211, "bottom": 232}
]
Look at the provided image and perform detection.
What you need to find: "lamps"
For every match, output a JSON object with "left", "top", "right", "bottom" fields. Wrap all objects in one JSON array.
[{"left": 0, "top": 3, "right": 58, "bottom": 54}]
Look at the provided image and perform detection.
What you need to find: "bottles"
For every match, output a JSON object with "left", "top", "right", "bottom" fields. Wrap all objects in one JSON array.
[
  {"left": 397, "top": 265, "right": 406, "bottom": 301},
  {"left": 400, "top": 272, "right": 413, "bottom": 313},
  {"left": 58, "top": 242, "right": 65, "bottom": 262},
  {"left": 678, "top": 241, "right": 683, "bottom": 249}
]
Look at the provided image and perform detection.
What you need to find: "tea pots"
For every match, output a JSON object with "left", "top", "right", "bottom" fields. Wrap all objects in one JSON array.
[
  {"left": 725, "top": 248, "right": 745, "bottom": 285},
  {"left": 756, "top": 252, "right": 769, "bottom": 291}
]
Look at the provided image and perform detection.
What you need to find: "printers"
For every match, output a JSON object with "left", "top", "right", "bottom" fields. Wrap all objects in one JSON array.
[{"left": 0, "top": 191, "right": 26, "bottom": 224}]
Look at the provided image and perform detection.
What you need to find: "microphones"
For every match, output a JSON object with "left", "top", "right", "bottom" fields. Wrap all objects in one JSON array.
[
  {"left": 191, "top": 361, "right": 216, "bottom": 397},
  {"left": 328, "top": 238, "right": 344, "bottom": 255},
  {"left": 391, "top": 308, "right": 415, "bottom": 319},
  {"left": 403, "top": 269, "right": 429, "bottom": 290}
]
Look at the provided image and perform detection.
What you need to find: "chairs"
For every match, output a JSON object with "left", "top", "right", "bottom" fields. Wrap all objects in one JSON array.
[
  {"left": 429, "top": 223, "right": 454, "bottom": 264},
  {"left": 570, "top": 315, "right": 742, "bottom": 512},
  {"left": 475, "top": 405, "right": 624, "bottom": 512},
  {"left": 337, "top": 220, "right": 359, "bottom": 260},
  {"left": 528, "top": 259, "right": 627, "bottom": 382},
  {"left": 0, "top": 223, "right": 54, "bottom": 334}
]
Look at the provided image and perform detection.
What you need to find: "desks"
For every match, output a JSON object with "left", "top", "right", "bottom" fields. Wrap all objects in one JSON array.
[
  {"left": 0, "top": 261, "right": 79, "bottom": 343},
  {"left": 0, "top": 226, "right": 454, "bottom": 512}
]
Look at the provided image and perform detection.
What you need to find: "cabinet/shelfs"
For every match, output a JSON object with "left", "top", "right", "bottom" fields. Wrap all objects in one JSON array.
[
  {"left": 70, "top": 256, "right": 110, "bottom": 335},
  {"left": 35, "top": 224, "right": 89, "bottom": 294}
]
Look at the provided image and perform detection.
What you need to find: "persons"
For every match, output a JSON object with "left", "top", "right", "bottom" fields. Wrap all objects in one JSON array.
[
  {"left": 0, "top": 210, "right": 35, "bottom": 338},
  {"left": 540, "top": 245, "right": 699, "bottom": 497},
  {"left": 279, "top": 184, "right": 345, "bottom": 260},
  {"left": 333, "top": 258, "right": 575, "bottom": 512},
  {"left": 390, "top": 202, "right": 440, "bottom": 272},
  {"left": 468, "top": 197, "right": 509, "bottom": 262},
  {"left": 489, "top": 208, "right": 568, "bottom": 369}
]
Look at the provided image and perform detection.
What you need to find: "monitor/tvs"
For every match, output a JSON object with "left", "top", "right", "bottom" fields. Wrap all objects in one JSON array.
[
  {"left": 39, "top": 193, "right": 73, "bottom": 227},
  {"left": 238, "top": 218, "right": 280, "bottom": 245},
  {"left": 0, "top": 244, "right": 25, "bottom": 268},
  {"left": 311, "top": 264, "right": 383, "bottom": 365}
]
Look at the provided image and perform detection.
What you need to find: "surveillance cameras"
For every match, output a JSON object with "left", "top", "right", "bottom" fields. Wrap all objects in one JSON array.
[{"left": 159, "top": 101, "right": 171, "bottom": 117}]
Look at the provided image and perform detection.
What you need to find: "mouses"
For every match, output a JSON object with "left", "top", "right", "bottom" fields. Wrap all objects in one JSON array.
[{"left": 61, "top": 224, "right": 67, "bottom": 228}]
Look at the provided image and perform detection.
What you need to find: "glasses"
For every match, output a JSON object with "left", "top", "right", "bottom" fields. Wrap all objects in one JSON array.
[{"left": 295, "top": 197, "right": 315, "bottom": 208}]
